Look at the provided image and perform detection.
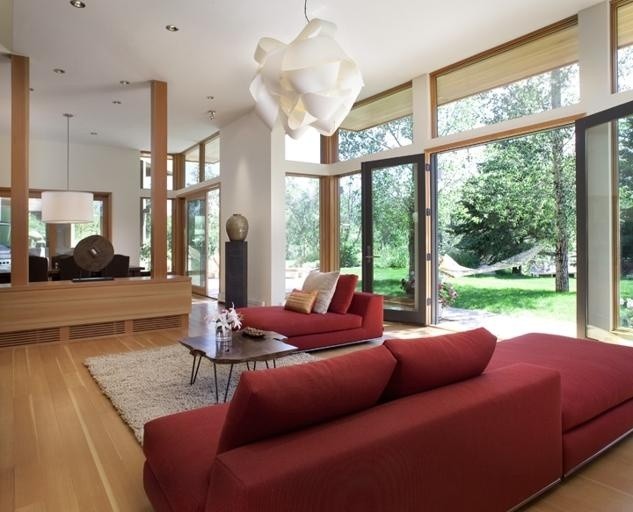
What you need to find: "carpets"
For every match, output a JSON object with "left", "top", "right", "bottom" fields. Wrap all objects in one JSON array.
[{"left": 81, "top": 341, "right": 324, "bottom": 447}]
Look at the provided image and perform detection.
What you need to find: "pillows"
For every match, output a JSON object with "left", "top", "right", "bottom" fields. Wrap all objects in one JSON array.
[
  {"left": 283, "top": 269, "right": 359, "bottom": 314},
  {"left": 384, "top": 327, "right": 498, "bottom": 396},
  {"left": 217, "top": 344, "right": 398, "bottom": 454}
]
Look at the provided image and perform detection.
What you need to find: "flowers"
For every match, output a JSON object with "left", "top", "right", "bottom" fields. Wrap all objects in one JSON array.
[
  {"left": 202, "top": 302, "right": 245, "bottom": 332},
  {"left": 402, "top": 263, "right": 458, "bottom": 306}
]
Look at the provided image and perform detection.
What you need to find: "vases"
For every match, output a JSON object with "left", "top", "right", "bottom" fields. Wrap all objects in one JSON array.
[
  {"left": 435, "top": 301, "right": 443, "bottom": 323},
  {"left": 213, "top": 330, "right": 235, "bottom": 342},
  {"left": 216, "top": 340, "right": 233, "bottom": 355},
  {"left": 226, "top": 214, "right": 249, "bottom": 241}
]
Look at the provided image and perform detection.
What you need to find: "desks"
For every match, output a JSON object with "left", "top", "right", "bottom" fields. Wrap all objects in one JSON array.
[{"left": 48, "top": 267, "right": 146, "bottom": 281}]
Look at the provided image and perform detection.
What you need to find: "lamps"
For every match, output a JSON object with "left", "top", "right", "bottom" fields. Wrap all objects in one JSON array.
[
  {"left": 41, "top": 111, "right": 94, "bottom": 224},
  {"left": 250, "top": 0, "right": 365, "bottom": 140}
]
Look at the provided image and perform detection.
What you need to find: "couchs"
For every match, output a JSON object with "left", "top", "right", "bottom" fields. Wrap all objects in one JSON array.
[{"left": 141, "top": 331, "right": 633, "bottom": 512}]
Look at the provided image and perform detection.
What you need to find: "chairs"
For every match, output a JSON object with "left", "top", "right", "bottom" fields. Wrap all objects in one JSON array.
[
  {"left": 29, "top": 255, "right": 48, "bottom": 282},
  {"left": 231, "top": 291, "right": 388, "bottom": 350},
  {"left": 52, "top": 254, "right": 68, "bottom": 281}
]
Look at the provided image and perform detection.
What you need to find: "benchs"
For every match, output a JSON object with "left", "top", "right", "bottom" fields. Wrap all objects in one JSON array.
[{"left": 60, "top": 254, "right": 130, "bottom": 281}]
